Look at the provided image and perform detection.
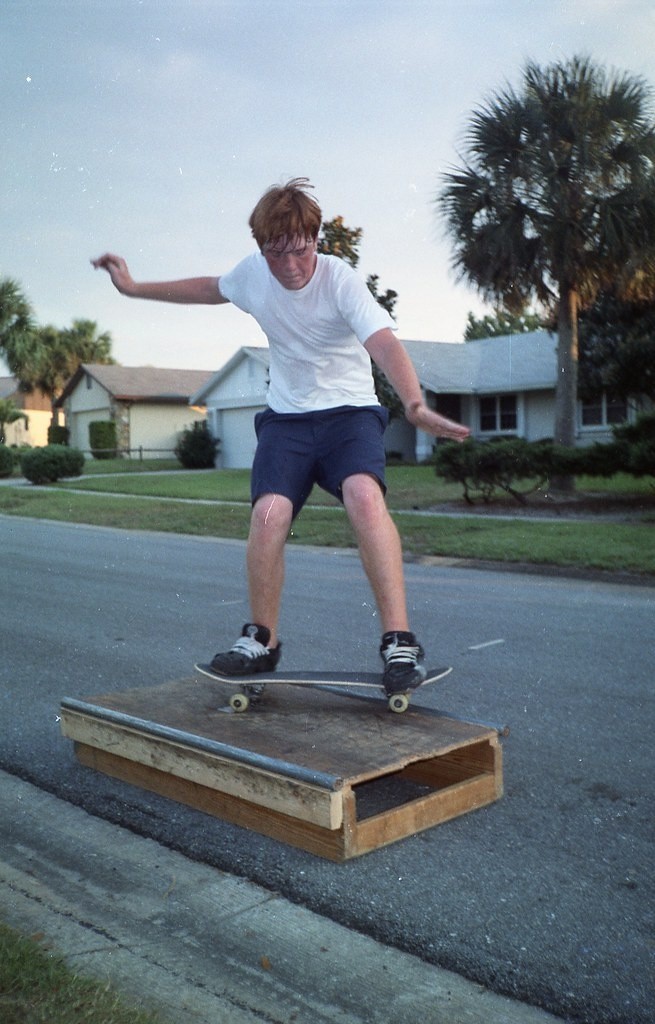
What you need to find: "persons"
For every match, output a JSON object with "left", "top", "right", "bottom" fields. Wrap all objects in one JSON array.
[{"left": 90, "top": 178, "right": 467, "bottom": 689}]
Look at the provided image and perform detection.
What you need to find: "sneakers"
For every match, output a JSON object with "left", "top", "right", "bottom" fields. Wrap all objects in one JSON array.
[
  {"left": 380, "top": 632, "right": 427, "bottom": 694},
  {"left": 210, "top": 623, "right": 283, "bottom": 676}
]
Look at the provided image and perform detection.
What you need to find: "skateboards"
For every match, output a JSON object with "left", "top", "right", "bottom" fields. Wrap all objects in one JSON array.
[{"left": 196, "top": 664, "right": 452, "bottom": 712}]
